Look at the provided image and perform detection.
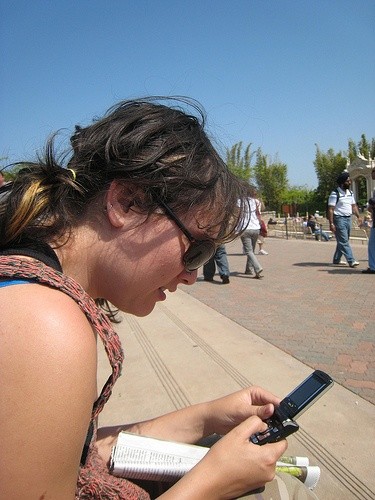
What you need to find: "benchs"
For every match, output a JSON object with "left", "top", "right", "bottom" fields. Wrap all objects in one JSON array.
[{"left": 268, "top": 224, "right": 315, "bottom": 240}]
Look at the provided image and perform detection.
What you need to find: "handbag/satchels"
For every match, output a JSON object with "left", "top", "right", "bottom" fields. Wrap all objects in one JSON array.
[
  {"left": 0, "top": 254, "right": 149, "bottom": 500},
  {"left": 256, "top": 200, "right": 267, "bottom": 237}
]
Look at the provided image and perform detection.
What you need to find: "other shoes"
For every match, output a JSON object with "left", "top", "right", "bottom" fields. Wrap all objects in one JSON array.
[
  {"left": 204, "top": 276, "right": 213, "bottom": 281},
  {"left": 338, "top": 261, "right": 346, "bottom": 265},
  {"left": 362, "top": 268, "right": 375, "bottom": 273},
  {"left": 350, "top": 262, "right": 360, "bottom": 266},
  {"left": 245, "top": 271, "right": 253, "bottom": 274},
  {"left": 222, "top": 273, "right": 229, "bottom": 283},
  {"left": 256, "top": 270, "right": 264, "bottom": 278}
]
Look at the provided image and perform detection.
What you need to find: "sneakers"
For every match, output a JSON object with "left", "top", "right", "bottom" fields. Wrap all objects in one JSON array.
[{"left": 258, "top": 249, "right": 268, "bottom": 255}]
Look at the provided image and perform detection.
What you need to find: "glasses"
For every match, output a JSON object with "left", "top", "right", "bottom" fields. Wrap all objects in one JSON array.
[{"left": 143, "top": 183, "right": 216, "bottom": 272}]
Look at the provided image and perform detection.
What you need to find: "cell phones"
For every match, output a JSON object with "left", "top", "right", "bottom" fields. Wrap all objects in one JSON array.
[{"left": 249, "top": 370, "right": 334, "bottom": 445}]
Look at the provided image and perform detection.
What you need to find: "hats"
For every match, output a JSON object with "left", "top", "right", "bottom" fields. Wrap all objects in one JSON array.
[{"left": 336, "top": 172, "right": 349, "bottom": 184}]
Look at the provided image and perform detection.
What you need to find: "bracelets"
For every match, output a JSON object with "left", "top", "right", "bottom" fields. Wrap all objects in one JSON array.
[{"left": 330, "top": 223, "right": 334, "bottom": 226}]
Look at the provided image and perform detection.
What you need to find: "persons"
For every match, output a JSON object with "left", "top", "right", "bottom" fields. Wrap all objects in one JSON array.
[
  {"left": 190, "top": 165, "right": 375, "bottom": 283},
  {"left": 0, "top": 94, "right": 288, "bottom": 500}
]
